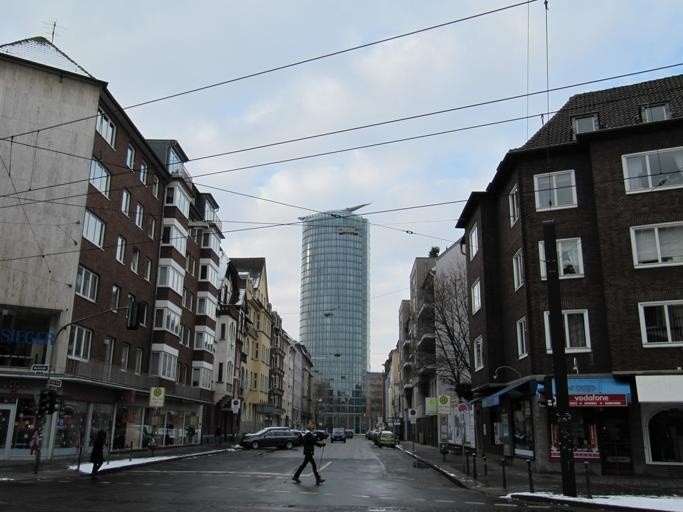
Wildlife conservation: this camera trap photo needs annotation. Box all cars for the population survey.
[365,426,400,447]
[330,427,353,442]
[232,423,329,449]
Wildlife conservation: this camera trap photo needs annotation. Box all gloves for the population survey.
[321,443,325,446]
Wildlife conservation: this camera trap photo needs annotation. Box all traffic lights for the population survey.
[538,376,553,410]
[37,388,62,416]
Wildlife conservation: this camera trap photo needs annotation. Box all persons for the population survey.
[292,425,326,484]
[29,430,41,455]
[89,430,108,481]
[215,424,223,446]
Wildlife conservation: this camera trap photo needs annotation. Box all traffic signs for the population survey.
[48,378,61,387]
[30,363,49,372]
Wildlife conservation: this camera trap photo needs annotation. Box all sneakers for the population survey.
[316,479,324,484]
[292,475,300,482]
